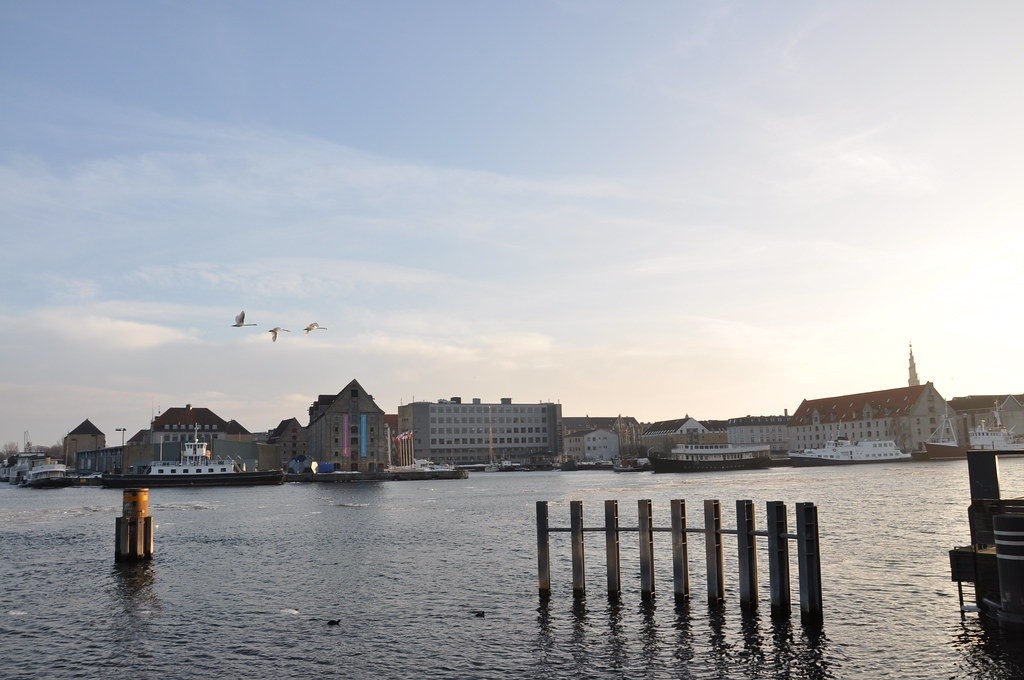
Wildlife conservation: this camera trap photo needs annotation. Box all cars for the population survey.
[91,473,103,478]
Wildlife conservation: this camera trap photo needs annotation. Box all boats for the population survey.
[968,398,1024,454]
[0,452,74,489]
[577,459,614,469]
[513,466,530,471]
[102,437,284,488]
[650,442,774,472]
[785,434,913,465]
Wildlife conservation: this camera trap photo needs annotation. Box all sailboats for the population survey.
[610,414,649,472]
[922,397,965,460]
[482,405,501,472]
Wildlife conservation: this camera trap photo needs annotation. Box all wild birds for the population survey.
[303,322,329,336]
[268,326,292,343]
[330,618,342,626]
[475,610,485,618]
[231,310,258,328]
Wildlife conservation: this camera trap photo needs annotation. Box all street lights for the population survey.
[92,435,98,453]
[72,438,78,451]
[116,428,126,446]
